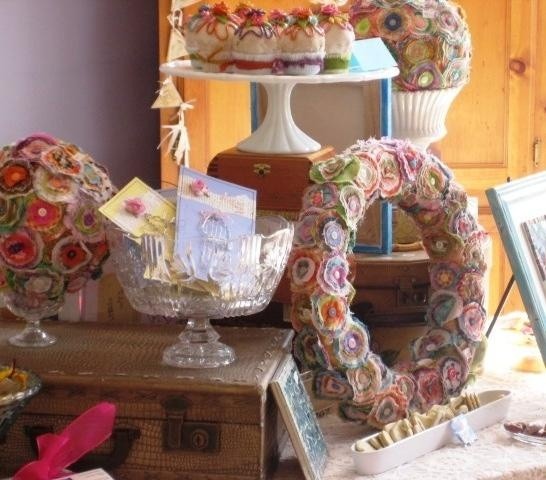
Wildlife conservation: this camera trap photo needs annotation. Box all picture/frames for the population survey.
[486,169,546,370]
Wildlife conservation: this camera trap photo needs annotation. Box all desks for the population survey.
[278,310,546,479]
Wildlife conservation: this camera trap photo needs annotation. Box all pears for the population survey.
[0,358,20,396]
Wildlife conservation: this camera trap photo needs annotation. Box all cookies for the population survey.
[355,391,481,451]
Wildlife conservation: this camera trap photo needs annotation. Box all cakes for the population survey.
[185,1,355,76]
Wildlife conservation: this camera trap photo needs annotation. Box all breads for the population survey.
[503,418,546,439]
